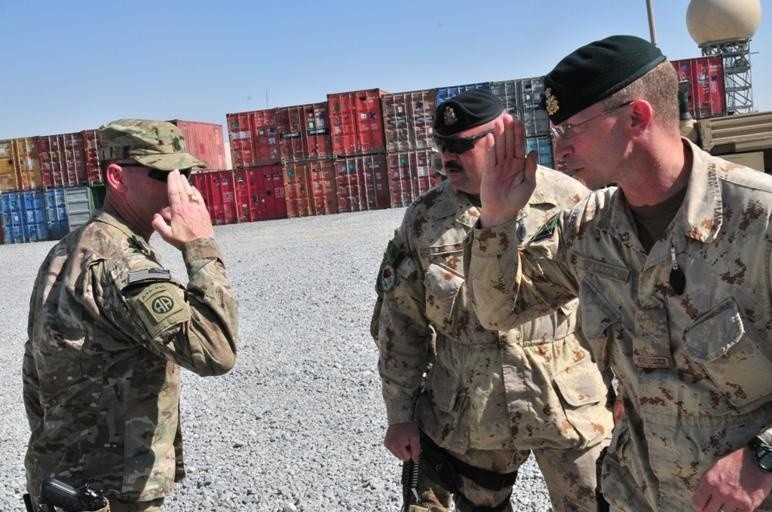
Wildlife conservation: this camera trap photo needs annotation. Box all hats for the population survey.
[97,117,207,172]
[538,35,667,127]
[434,87,503,136]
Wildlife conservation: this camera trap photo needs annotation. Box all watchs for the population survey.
[745,438,770,473]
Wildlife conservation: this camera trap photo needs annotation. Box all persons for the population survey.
[371,87,625,512]
[22,119,238,511]
[467,35,771,512]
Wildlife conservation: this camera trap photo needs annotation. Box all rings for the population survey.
[189,194,200,204]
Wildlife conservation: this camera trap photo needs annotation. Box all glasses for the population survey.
[111,163,192,182]
[546,99,634,142]
[431,128,496,156]
